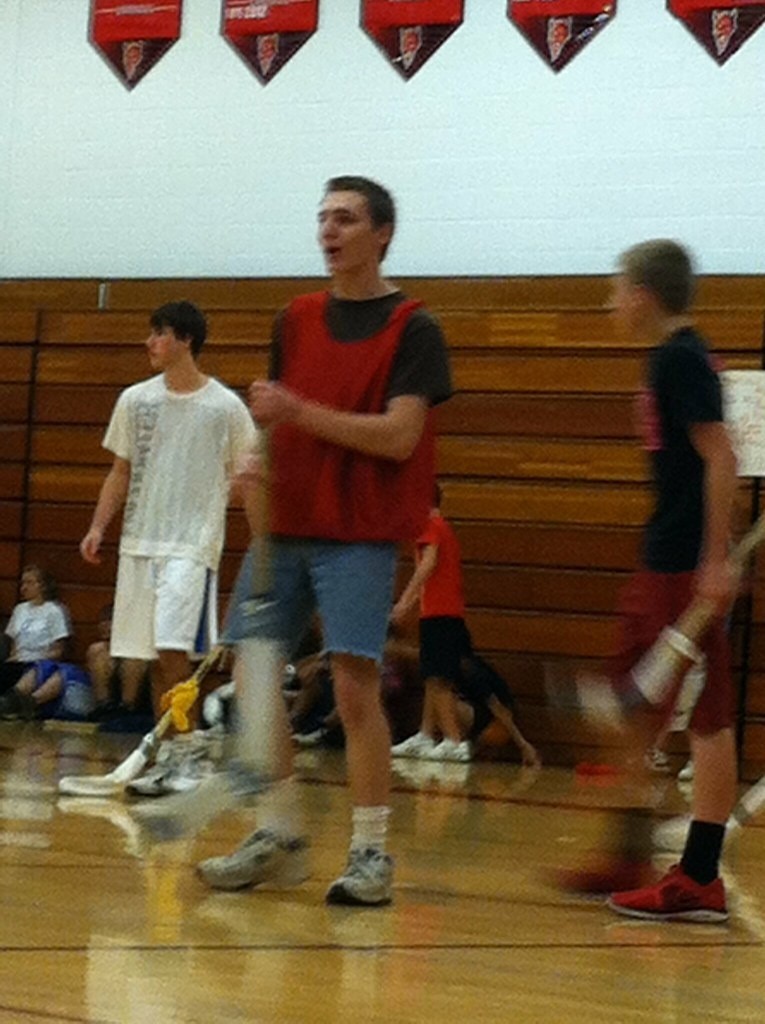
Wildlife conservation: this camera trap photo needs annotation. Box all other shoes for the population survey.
[12,685,35,720]
[0,698,17,719]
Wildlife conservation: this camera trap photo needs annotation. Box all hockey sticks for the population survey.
[60,646,229,797]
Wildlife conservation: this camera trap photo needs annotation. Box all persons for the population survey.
[193,174,457,908]
[538,240,743,923]
[0,479,541,768]
[81,298,263,799]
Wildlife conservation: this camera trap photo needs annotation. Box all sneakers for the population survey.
[428,741,470,761]
[198,828,312,890]
[389,737,434,757]
[326,848,393,905]
[608,864,730,921]
[163,730,228,790]
[124,739,175,796]
[556,859,651,900]
